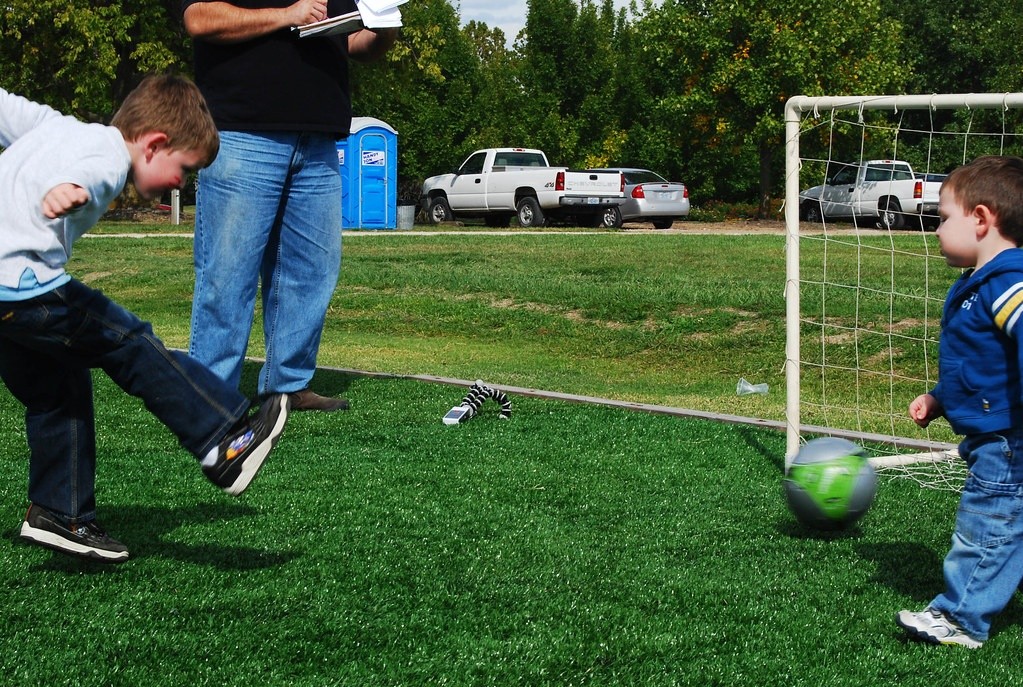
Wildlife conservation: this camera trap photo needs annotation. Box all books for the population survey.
[296,10,363,39]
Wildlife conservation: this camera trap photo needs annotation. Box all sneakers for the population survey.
[202,392,292,497]
[19,503,130,562]
[895,607,983,651]
[290,388,348,410]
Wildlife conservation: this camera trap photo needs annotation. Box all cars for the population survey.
[547,168,690,229]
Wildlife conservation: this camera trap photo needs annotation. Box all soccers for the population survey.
[786,436,876,529]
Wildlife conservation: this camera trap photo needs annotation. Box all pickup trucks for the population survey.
[419,148,628,228]
[799,160,950,230]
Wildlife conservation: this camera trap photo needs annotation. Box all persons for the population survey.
[894,154,1022,649]
[0,73,287,563]
[178,0,401,413]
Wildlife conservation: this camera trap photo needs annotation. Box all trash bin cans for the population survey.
[335,117,399,229]
